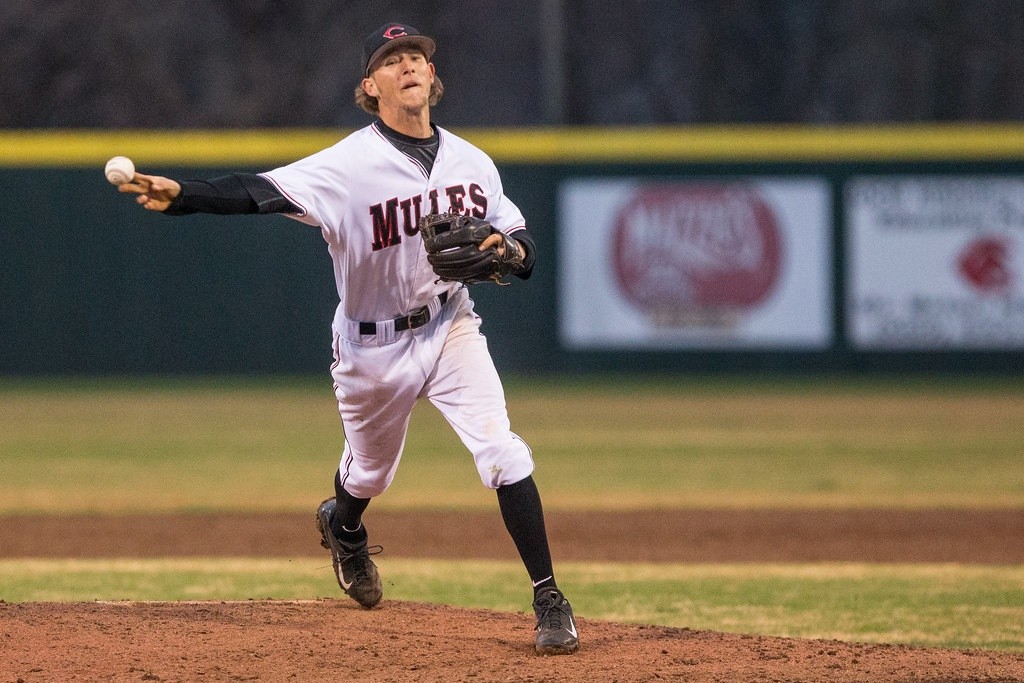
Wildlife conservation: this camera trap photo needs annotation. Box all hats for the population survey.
[361,22,435,78]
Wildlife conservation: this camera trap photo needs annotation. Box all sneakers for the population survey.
[318,497,381,609]
[533,587,579,655]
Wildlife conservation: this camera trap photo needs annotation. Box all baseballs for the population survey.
[105,155,136,186]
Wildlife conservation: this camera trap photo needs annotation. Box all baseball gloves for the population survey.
[418,212,521,286]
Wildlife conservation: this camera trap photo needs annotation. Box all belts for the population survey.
[359,291,447,334]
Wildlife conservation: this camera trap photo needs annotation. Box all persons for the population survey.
[120,23,580,655]
